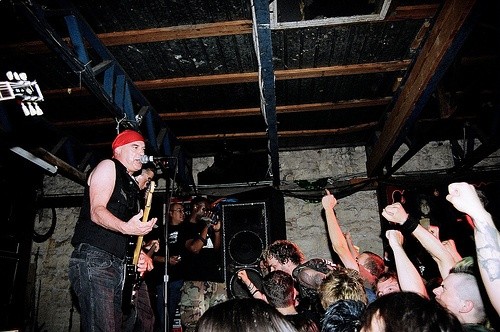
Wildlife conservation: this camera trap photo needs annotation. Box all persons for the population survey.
[196,182,500,332]
[151,197,226,332]
[68,130,159,332]
[177,197,228,332]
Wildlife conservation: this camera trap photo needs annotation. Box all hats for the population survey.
[112,129,144,149]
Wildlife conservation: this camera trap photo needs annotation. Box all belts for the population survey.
[76,237,124,260]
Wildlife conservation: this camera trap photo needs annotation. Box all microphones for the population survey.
[140,155,177,164]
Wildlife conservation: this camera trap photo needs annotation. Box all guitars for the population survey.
[137,237,160,290]
[121,180,156,316]
[0,70,46,117]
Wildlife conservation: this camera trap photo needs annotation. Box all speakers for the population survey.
[222,201,287,300]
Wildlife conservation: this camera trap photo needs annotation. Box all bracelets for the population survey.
[403,214,418,233]
[249,285,255,291]
[251,290,259,295]
[215,229,220,232]
[196,234,208,246]
[247,282,251,287]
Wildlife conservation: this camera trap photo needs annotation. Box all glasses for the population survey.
[170,208,185,212]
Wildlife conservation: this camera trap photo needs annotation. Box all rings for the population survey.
[444,243,446,245]
[176,260,178,263]
